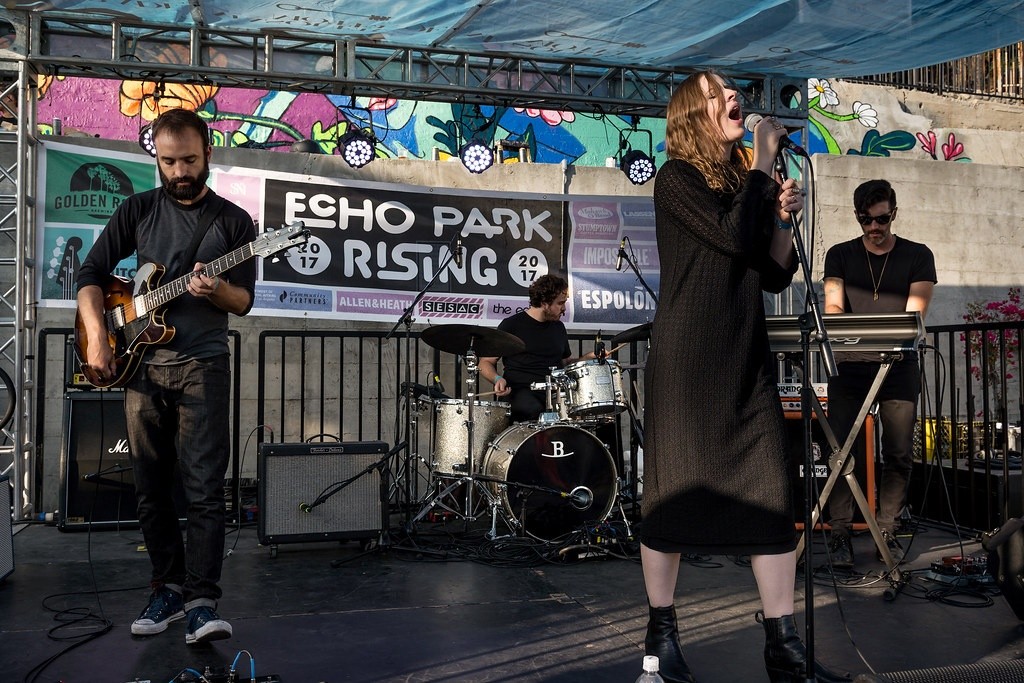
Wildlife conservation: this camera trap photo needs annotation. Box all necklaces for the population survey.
[866,239,892,302]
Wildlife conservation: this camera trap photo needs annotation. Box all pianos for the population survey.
[763,310,928,586]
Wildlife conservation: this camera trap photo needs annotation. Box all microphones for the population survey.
[572,490,591,507]
[456,232,462,269]
[616,238,626,271]
[593,331,601,355]
[80,473,96,481]
[298,503,311,513]
[433,371,446,393]
[743,113,810,158]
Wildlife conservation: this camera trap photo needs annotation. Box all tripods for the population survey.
[328,250,516,569]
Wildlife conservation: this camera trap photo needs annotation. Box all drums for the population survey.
[431,398,514,480]
[478,420,620,545]
[555,378,615,427]
[557,357,628,420]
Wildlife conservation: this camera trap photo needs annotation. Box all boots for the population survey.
[645,605,697,683]
[753,608,854,683]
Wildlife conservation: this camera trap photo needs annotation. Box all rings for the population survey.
[791,195,799,202]
[792,178,797,182]
[791,187,800,195]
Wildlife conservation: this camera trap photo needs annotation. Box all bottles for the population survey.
[635,656,665,683]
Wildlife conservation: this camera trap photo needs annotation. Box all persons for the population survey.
[637,69,854,683]
[477,274,609,427]
[823,179,938,567]
[75,108,256,645]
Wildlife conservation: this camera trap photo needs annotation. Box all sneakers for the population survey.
[186,606,233,645]
[876,531,907,562]
[130,587,187,634]
[827,533,855,565]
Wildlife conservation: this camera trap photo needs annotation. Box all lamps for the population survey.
[458,105,496,175]
[336,93,377,169]
[138,82,183,158]
[619,115,656,186]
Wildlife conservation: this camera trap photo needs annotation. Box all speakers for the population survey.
[0,474,15,581]
[258,441,389,546]
[57,394,189,533]
[853,657,1024,683]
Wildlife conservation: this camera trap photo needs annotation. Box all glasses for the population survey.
[856,209,894,225]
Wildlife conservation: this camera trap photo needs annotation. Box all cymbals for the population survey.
[610,321,653,343]
[420,323,528,359]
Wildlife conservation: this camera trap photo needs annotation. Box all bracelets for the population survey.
[775,215,792,230]
[493,375,502,385]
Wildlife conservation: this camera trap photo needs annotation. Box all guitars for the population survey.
[74,220,311,390]
